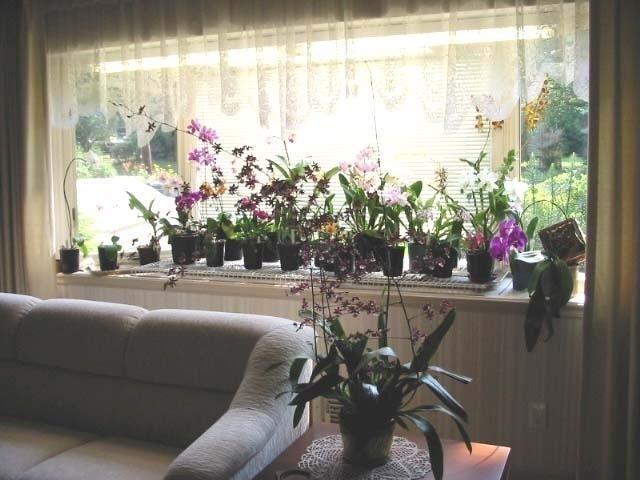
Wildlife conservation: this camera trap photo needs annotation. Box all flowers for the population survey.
[203,228,223,242]
[172,191,209,235]
[406,190,438,246]
[490,219,527,264]
[307,168,344,243]
[377,178,413,244]
[200,179,242,240]
[102,98,472,480]
[337,145,411,237]
[430,166,471,248]
[426,72,551,253]
[266,129,301,246]
[212,143,273,241]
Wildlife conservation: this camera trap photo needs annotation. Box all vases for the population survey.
[509,255,527,290]
[333,408,396,468]
[314,251,335,273]
[276,241,300,271]
[205,242,225,268]
[380,243,405,277]
[241,240,265,270]
[408,242,432,275]
[354,229,380,270]
[223,239,243,261]
[431,245,456,278]
[466,250,493,284]
[171,234,202,264]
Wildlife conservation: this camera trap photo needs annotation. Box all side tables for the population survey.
[254,421,513,480]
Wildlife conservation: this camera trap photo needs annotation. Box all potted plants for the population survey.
[126,187,166,265]
[519,164,586,264]
[58,235,89,274]
[97,234,121,272]
[524,236,579,354]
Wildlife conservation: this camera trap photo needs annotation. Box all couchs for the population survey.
[2,290,316,480]
